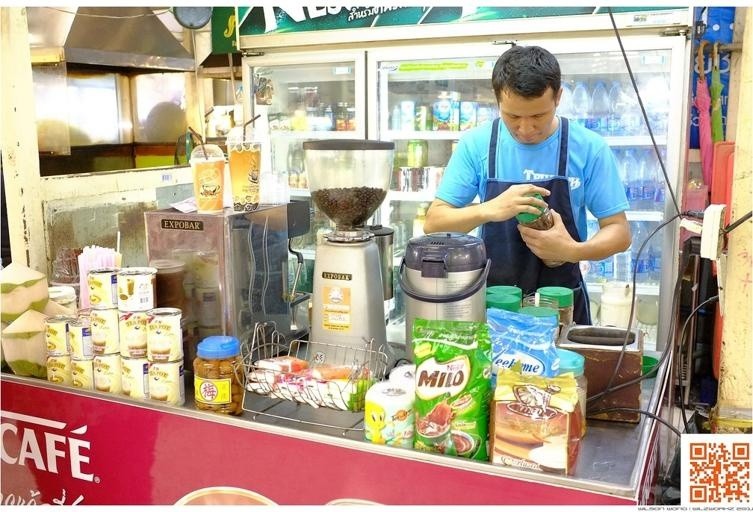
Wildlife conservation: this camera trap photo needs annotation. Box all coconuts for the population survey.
[1,262,75,378]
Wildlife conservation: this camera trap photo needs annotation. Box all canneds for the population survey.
[516,193,566,268]
[397,139,437,192]
[44,267,246,416]
[486,285,575,317]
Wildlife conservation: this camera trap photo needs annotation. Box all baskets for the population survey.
[230,320,387,437]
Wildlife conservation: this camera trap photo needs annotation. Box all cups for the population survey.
[190,129,264,214]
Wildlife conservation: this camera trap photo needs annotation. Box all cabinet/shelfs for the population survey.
[368,32,692,349]
[240,50,367,262]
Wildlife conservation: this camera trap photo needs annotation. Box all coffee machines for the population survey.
[303,138,392,376]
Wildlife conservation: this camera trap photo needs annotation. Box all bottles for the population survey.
[486,79,667,343]
[391,104,401,129]
[412,208,428,237]
[287,142,304,185]
[288,87,355,131]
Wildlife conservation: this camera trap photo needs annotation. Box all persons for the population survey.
[423,46,630,325]
